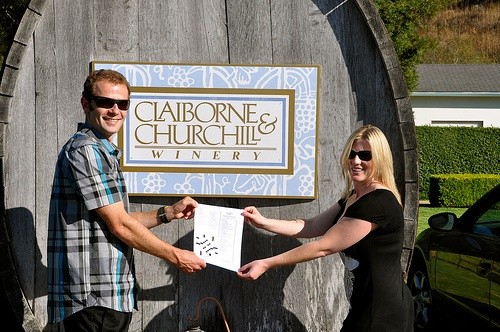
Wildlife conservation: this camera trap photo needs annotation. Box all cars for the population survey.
[406,182,500,332]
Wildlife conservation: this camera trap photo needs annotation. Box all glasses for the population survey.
[89,95,131,110]
[348,150,372,161]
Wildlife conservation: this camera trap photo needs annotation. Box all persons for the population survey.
[48,69,206,331]
[237,125,415,332]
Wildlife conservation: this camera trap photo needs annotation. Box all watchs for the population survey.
[158,206,171,224]
